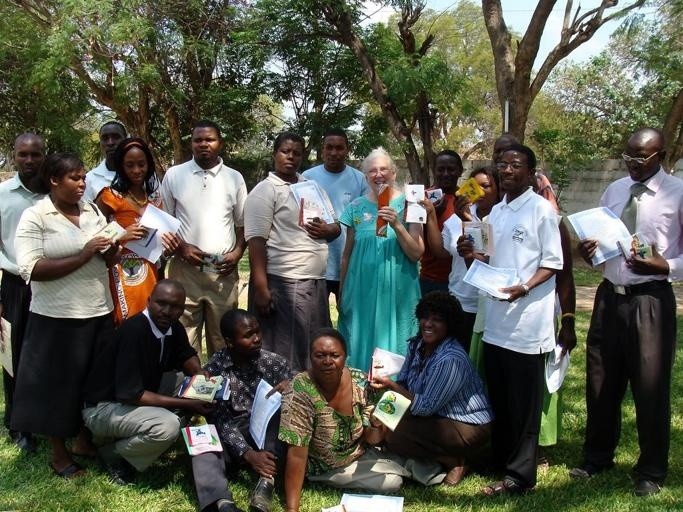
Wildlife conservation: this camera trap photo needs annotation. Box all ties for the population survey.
[620,183,647,233]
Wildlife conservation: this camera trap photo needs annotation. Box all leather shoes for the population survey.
[537,452,549,472]
[249,478,276,512]
[216,500,238,511]
[567,462,609,480]
[633,478,663,497]
[108,462,138,485]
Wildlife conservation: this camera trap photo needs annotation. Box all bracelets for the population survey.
[561,313,576,319]
[522,284,529,295]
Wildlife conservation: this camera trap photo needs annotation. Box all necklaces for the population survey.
[122,182,149,207]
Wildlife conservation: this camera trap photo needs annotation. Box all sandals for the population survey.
[475,479,531,498]
[443,463,464,485]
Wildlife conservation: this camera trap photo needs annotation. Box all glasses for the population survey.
[620,147,659,167]
[494,160,532,171]
[363,165,394,176]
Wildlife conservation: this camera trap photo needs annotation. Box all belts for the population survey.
[602,278,658,297]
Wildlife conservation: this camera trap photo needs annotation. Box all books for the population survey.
[181,424,224,456]
[90,221,127,254]
[617,231,654,263]
[462,221,494,258]
[178,375,231,403]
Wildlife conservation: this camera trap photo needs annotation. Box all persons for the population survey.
[338,147,426,454]
[367,291,495,487]
[454,133,576,469]
[83,120,128,202]
[83,279,232,487]
[417,166,502,353]
[10,154,121,476]
[302,127,373,293]
[182,309,294,512]
[277,326,448,512]
[161,121,248,367]
[244,132,342,372]
[0,134,45,452]
[419,150,462,297]
[456,144,564,497]
[569,128,683,496]
[93,138,183,334]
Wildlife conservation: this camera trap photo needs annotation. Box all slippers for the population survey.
[71,442,98,458]
[48,458,87,480]
